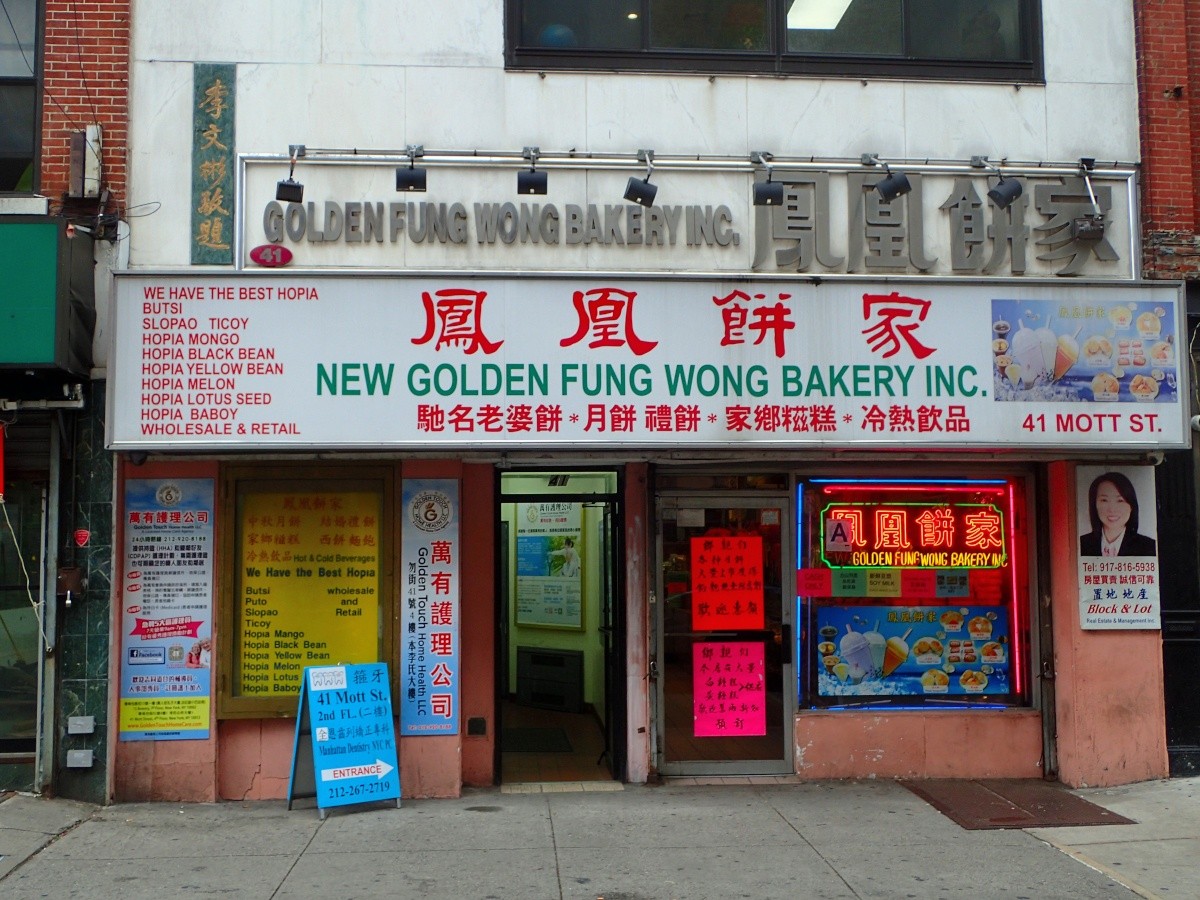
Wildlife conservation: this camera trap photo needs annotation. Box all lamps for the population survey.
[396,145,427,191]
[518,147,548,194]
[860,154,912,204]
[749,152,783,205]
[1071,163,1104,239]
[624,150,658,206]
[276,145,307,203]
[971,156,1022,208]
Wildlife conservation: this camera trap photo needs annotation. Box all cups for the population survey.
[817,626,907,687]
[992,321,1075,392]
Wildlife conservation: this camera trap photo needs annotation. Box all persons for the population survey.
[1080,472,1156,557]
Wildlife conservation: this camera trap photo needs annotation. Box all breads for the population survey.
[969,616,990,633]
[1083,340,1113,357]
[1130,375,1157,395]
[1091,373,1119,395]
[960,670,985,685]
[921,669,948,686]
[914,640,941,654]
